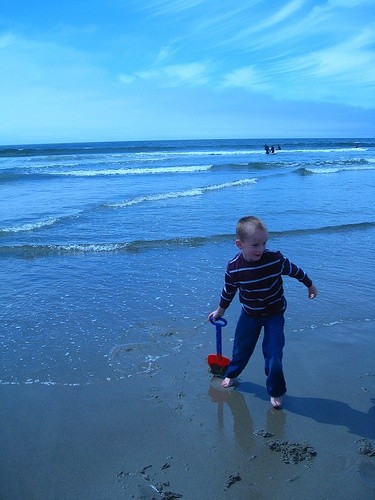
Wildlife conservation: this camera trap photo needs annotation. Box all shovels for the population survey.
[207,317,232,375]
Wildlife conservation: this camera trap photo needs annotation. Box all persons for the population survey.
[264,144,269,154]
[276,144,281,150]
[208,216,317,406]
[271,144,274,153]
